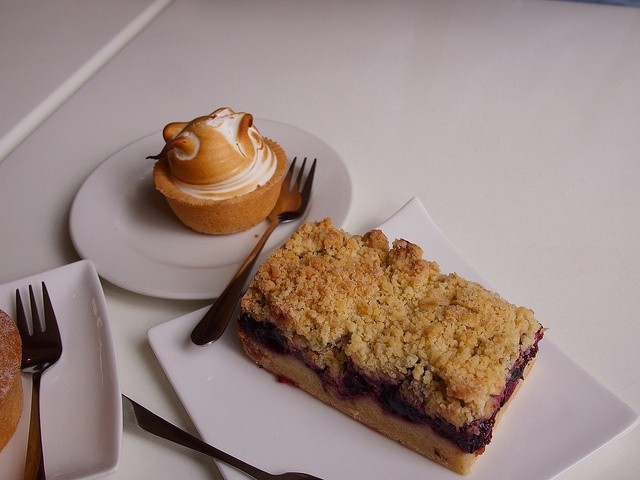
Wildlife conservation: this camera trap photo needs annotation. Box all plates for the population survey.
[0,259,123,480]
[68,116,352,301]
[145,196,640,480]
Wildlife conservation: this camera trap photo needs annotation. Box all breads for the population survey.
[235,217,549,477]
[0,307,24,454]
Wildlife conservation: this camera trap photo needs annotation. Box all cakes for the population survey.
[144,105,288,235]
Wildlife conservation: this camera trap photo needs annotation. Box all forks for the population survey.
[190,156,317,348]
[16,281,62,480]
